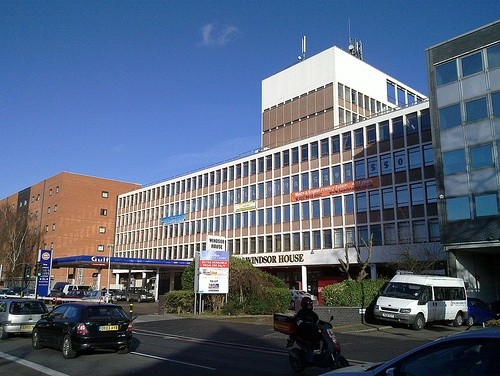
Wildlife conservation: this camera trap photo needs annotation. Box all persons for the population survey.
[294,297,323,362]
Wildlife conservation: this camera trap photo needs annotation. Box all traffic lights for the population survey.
[34,263,43,277]
[24,263,32,279]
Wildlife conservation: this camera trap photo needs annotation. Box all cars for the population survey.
[288,289,318,310]
[0,286,27,306]
[318,326,500,376]
[125,287,155,303]
[21,289,36,297]
[52,282,95,299]
[466,297,498,327]
[32,302,133,360]
[101,288,127,301]
[0,298,49,340]
[44,290,66,304]
[82,290,117,304]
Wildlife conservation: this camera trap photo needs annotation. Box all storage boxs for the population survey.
[274,313,297,333]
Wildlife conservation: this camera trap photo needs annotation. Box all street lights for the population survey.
[107,244,114,293]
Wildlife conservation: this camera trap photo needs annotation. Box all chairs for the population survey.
[92,309,102,315]
[24,303,31,309]
[13,304,21,310]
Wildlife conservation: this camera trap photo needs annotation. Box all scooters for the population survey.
[285,315,350,374]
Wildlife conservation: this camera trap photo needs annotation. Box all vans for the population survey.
[373,274,469,331]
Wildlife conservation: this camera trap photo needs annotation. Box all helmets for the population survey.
[300,297,313,311]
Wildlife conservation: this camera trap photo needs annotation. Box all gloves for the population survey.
[305,322,314,328]
[324,322,332,329]
